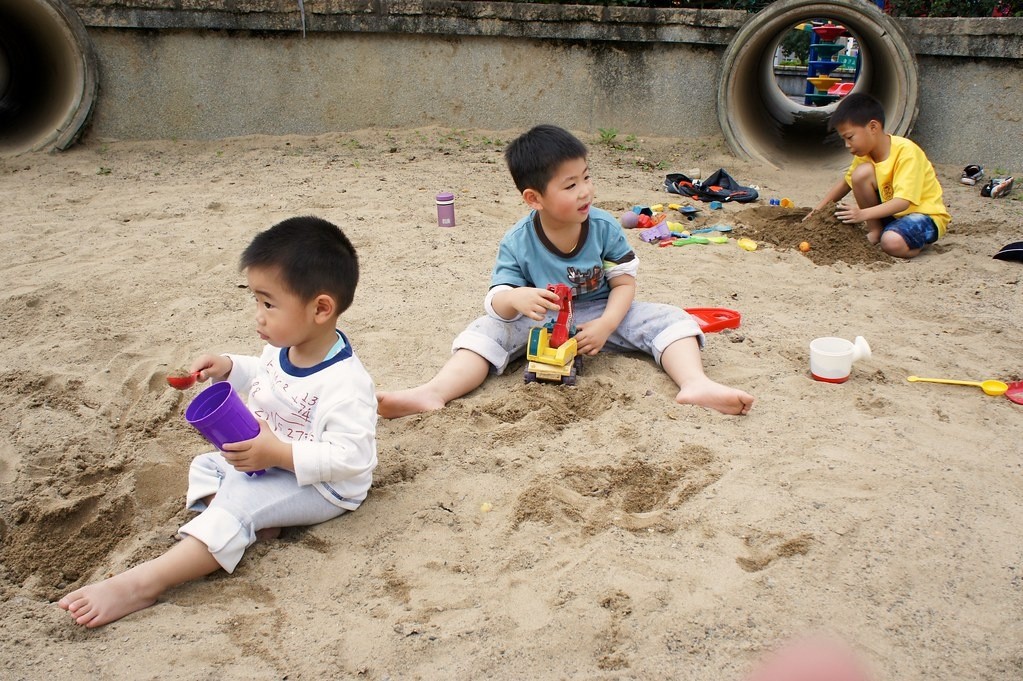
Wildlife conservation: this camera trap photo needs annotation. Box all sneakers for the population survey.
[959,163,983,185]
[982,177,1014,198]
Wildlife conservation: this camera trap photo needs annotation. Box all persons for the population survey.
[802,93,953,258]
[58,216,378,630]
[376,124,755,418]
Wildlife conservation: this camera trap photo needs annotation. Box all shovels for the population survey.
[671,238,709,247]
[692,224,732,234]
[668,202,703,215]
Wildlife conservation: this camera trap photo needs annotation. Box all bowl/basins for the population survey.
[810,337,853,383]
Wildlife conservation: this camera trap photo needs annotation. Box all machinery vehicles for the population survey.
[522,281,583,385]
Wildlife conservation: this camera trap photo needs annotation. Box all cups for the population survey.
[186,381,276,477]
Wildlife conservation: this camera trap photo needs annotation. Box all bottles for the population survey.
[436,193,456,227]
